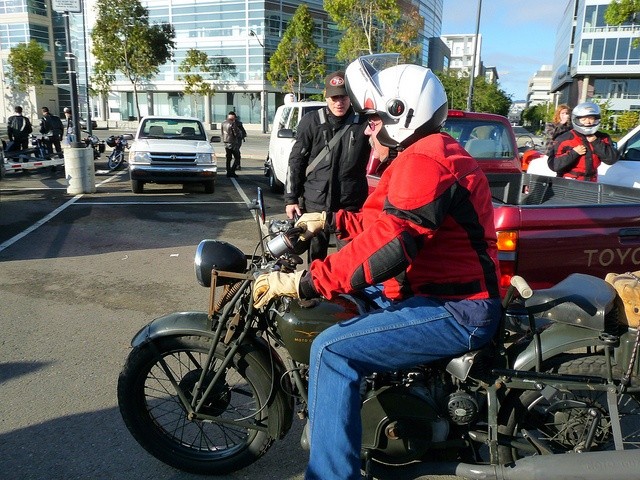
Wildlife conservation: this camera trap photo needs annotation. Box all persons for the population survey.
[37,107,63,159]
[229,111,247,169]
[547,101,616,181]
[222,114,245,176]
[64,106,76,132]
[547,104,569,155]
[7,105,31,163]
[286,72,370,266]
[254,51,504,480]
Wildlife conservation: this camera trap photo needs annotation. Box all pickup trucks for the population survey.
[78,117,97,128]
[363,109,640,299]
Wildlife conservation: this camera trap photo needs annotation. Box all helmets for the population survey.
[345,53,448,150]
[106,137,115,147]
[571,103,601,135]
[267,218,310,256]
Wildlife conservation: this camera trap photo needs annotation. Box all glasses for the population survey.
[366,116,382,129]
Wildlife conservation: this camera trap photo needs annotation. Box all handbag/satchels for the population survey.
[606,269,640,329]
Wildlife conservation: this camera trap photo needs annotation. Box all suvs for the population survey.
[265,100,329,193]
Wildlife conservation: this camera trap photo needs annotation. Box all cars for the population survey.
[122,116,222,193]
[527,124,640,188]
[510,126,546,148]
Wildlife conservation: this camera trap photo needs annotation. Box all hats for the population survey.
[325,71,347,97]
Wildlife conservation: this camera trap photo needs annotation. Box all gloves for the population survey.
[294,211,326,241]
[251,269,307,309]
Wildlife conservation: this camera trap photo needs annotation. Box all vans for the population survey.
[60,119,72,128]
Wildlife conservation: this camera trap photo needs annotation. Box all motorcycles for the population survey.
[106,134,131,171]
[29,129,53,160]
[116,186,640,479]
[82,129,106,160]
